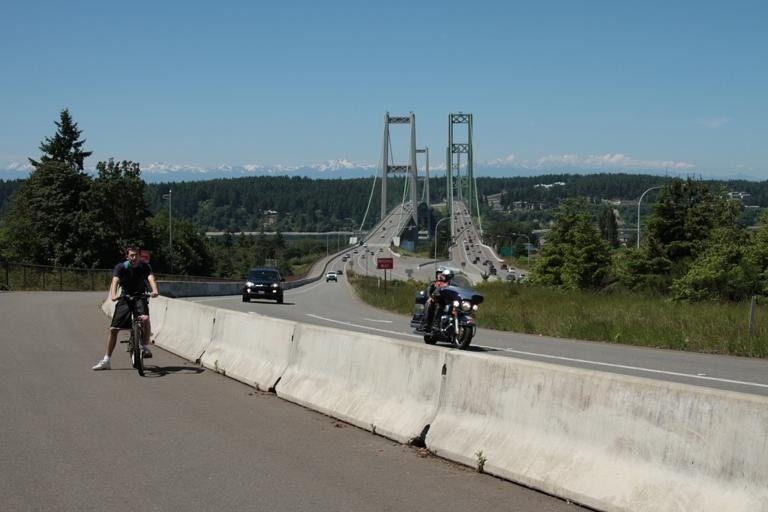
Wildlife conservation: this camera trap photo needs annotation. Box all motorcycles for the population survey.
[410,284,484,350]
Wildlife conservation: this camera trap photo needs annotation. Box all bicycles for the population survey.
[117,291,152,377]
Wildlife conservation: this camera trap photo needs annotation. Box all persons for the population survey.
[415,267,446,334]
[91,244,160,370]
[431,269,456,328]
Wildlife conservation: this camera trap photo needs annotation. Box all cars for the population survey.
[242,267,285,303]
[489,264,525,280]
[326,270,337,282]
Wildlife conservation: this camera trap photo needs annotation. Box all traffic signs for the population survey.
[376,258,392,269]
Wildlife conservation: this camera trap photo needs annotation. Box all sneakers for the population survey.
[92,360,111,370]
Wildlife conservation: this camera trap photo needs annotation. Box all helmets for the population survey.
[436,267,454,275]
